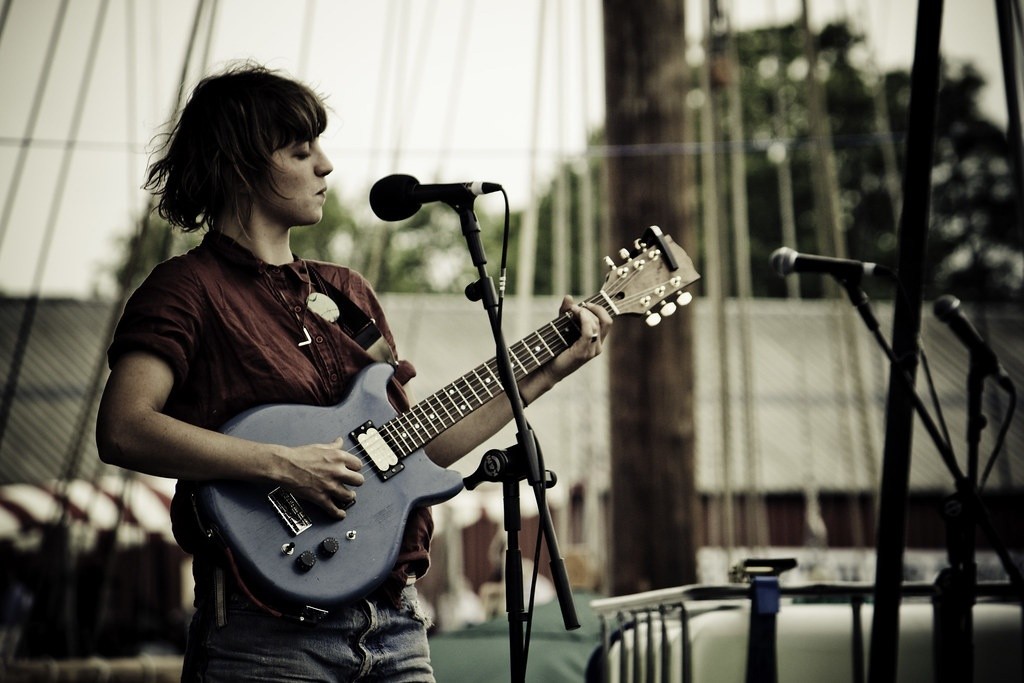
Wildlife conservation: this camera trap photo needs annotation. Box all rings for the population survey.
[591,334,597,343]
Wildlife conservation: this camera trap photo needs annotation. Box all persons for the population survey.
[96,73,614,681]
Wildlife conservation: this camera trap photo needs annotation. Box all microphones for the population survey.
[370,174,503,222]
[933,295,1016,395]
[770,247,879,276]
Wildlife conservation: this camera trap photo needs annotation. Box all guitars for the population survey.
[186,223,706,628]
